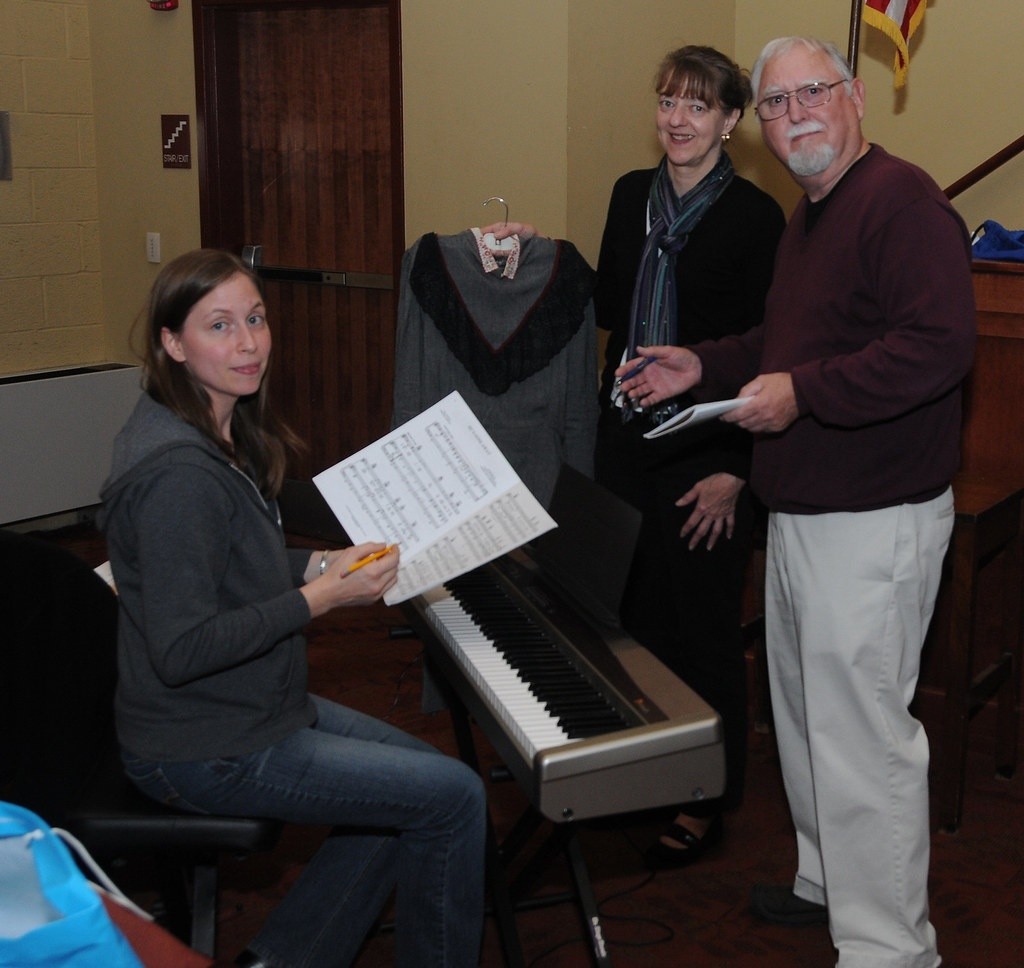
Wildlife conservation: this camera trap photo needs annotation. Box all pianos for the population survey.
[402,543,728,825]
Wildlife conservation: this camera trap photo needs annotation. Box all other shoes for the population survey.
[645,814,724,865]
[752,886,827,926]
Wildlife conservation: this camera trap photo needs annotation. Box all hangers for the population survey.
[482,196,517,257]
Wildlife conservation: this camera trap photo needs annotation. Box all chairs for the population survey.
[0,503,280,962]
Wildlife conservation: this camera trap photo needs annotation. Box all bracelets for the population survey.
[320,547,331,574]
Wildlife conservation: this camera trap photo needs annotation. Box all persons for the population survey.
[99,246,494,968]
[613,29,981,967]
[478,45,791,869]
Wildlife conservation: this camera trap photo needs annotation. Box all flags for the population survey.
[860,0,928,91]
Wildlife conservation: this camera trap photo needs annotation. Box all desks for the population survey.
[745,260,1024,845]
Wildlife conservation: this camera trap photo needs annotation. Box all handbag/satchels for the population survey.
[969,220,1024,261]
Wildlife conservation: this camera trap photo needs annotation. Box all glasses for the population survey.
[754,78,850,120]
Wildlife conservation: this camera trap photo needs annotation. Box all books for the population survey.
[643,395,756,441]
